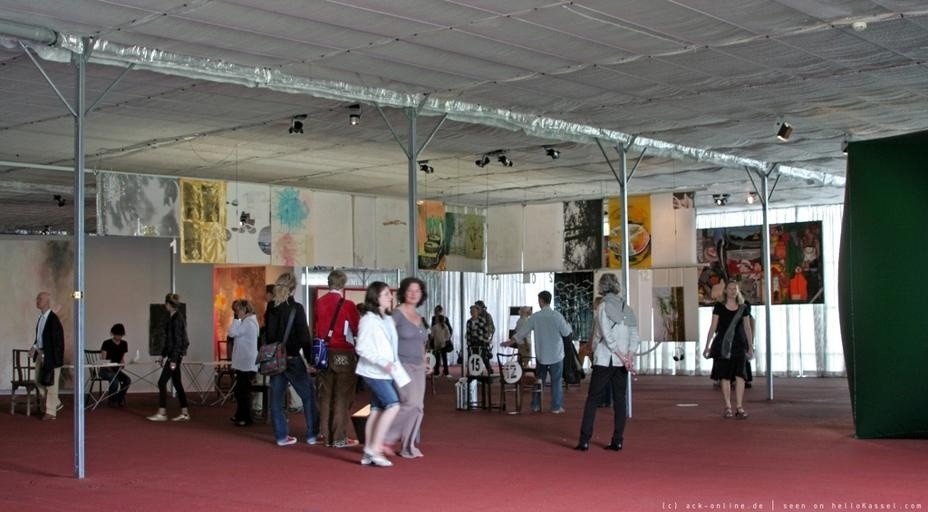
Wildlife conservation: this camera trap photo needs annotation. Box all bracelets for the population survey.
[623,357,626,363]
[748,344,754,349]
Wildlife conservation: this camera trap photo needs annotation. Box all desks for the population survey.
[60,361,127,411]
[127,356,240,410]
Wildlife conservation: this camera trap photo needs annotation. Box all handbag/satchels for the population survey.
[707,326,735,360]
[446,340,454,352]
[255,341,288,375]
[37,365,55,385]
[310,338,329,370]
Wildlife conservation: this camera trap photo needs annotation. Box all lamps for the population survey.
[415,136,565,174]
[284,113,309,137]
[347,110,363,128]
[707,120,850,209]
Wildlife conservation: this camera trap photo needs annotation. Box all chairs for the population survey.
[83,349,127,408]
[461,338,546,414]
[216,340,240,401]
[10,349,45,420]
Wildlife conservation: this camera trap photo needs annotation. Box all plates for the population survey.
[608,222,649,256]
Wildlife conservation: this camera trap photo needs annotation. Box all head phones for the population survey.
[673,346,685,362]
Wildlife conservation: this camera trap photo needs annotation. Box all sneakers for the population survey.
[170,413,191,421]
[146,414,168,421]
[40,413,56,422]
[277,436,297,446]
[307,434,324,444]
[362,447,393,467]
[360,455,372,464]
[324,440,333,447]
[332,438,359,448]
[56,403,64,411]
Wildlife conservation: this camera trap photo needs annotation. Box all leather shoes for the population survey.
[574,443,589,451]
[604,442,622,451]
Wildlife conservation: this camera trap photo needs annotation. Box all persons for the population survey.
[146,293,192,423]
[729,300,755,389]
[99,323,130,408]
[28,292,64,422]
[227,268,573,469]
[702,280,756,420]
[572,270,639,452]
[585,297,612,407]
